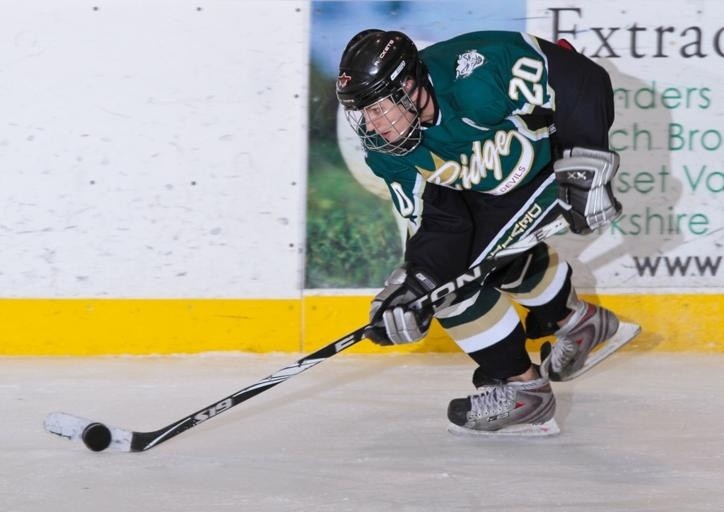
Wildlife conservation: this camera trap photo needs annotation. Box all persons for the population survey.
[335,28,623,433]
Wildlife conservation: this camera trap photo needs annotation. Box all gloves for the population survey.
[365,262,447,346]
[553,147,623,235]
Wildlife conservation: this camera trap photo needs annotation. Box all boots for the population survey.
[447,286,620,432]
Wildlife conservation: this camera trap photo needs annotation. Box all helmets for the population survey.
[336,29,428,110]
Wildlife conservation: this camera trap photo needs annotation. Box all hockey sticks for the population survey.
[48,216,570,452]
